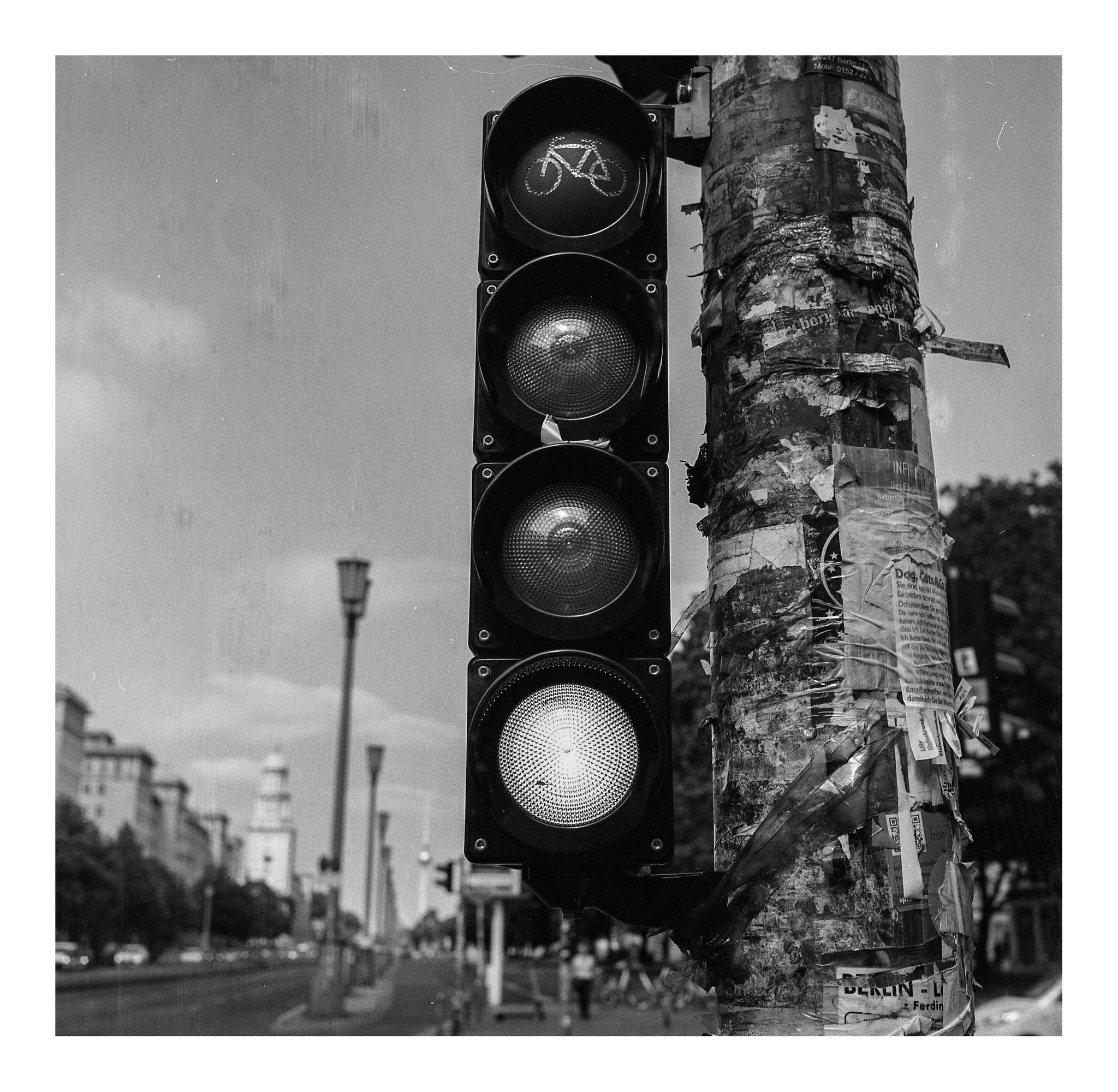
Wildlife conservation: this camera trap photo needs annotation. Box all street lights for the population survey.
[315,556,376,1018]
[360,742,397,981]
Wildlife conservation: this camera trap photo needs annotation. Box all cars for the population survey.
[113,944,149,966]
[178,946,207,965]
[55,941,87,972]
[973,971,1063,1036]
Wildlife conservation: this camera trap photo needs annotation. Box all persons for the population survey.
[571,941,596,1021]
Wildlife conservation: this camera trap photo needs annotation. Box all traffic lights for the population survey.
[435,861,454,891]
[461,73,675,870]
